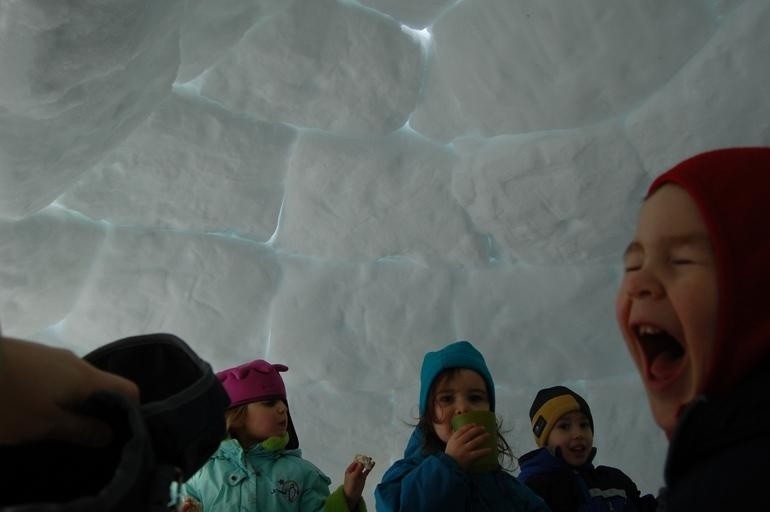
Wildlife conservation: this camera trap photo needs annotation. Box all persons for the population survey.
[514,385,657,512]
[614,146,769,512]
[0,335,141,447]
[373,340,552,512]
[170,359,376,512]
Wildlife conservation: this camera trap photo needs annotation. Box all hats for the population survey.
[416,338,497,418]
[527,385,592,452]
[213,360,300,452]
[639,144,770,433]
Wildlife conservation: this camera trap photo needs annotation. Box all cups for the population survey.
[452,412,499,465]
